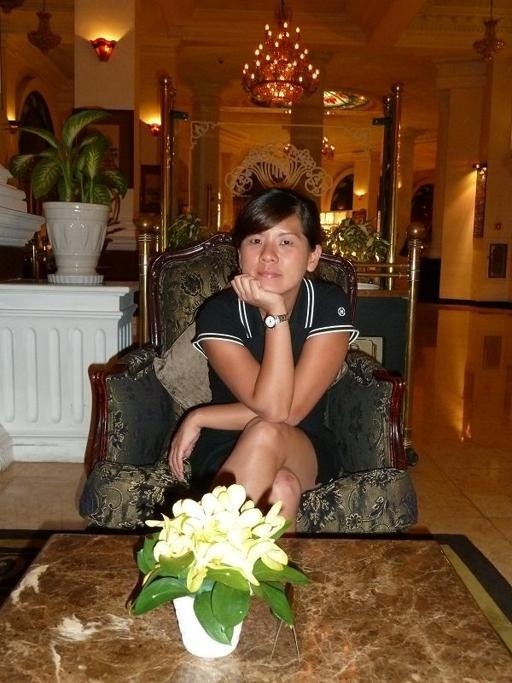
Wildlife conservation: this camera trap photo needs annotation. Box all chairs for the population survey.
[79,230,418,535]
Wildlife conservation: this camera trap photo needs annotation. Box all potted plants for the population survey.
[6,107,127,285]
[323,218,392,290]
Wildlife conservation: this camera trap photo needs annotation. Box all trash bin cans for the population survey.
[419,257,441,304]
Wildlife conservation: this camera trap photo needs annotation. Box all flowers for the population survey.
[128,484,310,645]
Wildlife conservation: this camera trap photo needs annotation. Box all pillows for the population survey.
[152,321,211,409]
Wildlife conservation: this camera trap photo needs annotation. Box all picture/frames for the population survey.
[139,164,162,214]
[488,242,508,278]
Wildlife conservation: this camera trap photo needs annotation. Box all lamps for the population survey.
[90,37,118,63]
[239,0,320,109]
[26,0,62,57]
[472,0,505,64]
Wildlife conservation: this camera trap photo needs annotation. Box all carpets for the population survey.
[0,520,512,683]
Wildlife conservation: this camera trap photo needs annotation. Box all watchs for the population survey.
[260,313,288,329]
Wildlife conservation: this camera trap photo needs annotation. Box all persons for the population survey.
[168,186,359,536]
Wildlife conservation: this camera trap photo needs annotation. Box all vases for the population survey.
[171,596,244,657]
